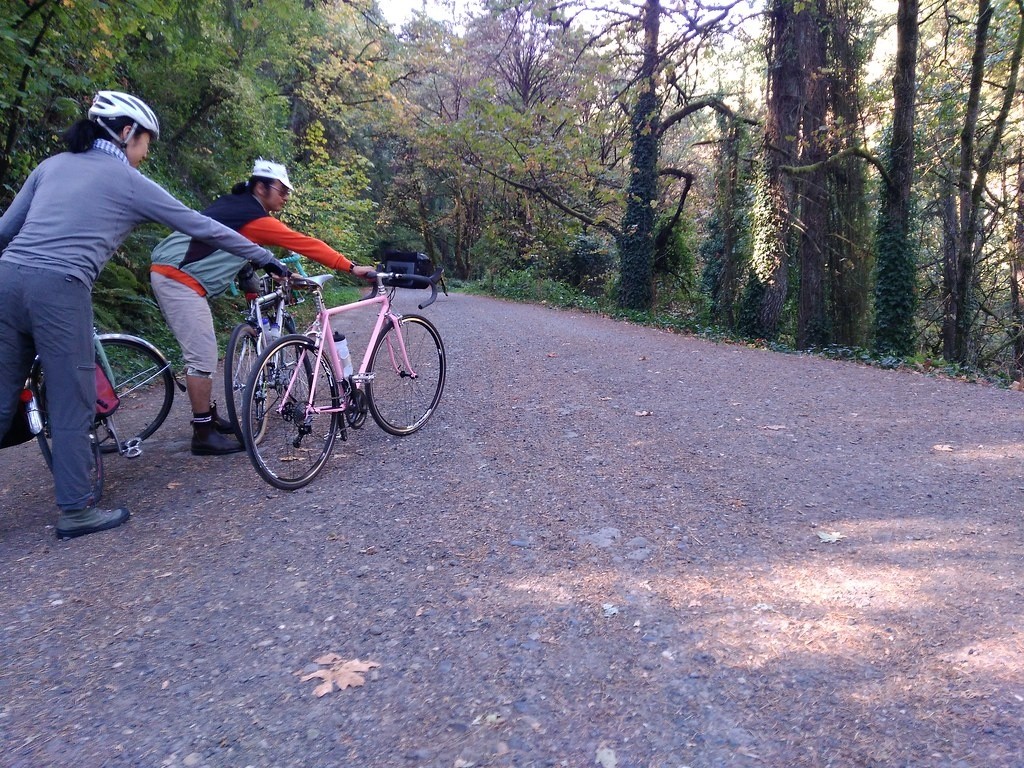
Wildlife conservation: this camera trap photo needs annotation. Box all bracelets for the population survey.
[349,264,354,273]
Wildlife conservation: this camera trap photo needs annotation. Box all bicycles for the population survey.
[222,257,320,448]
[239,266,449,490]
[22,321,188,510]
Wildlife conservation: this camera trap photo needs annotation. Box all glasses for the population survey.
[269,184,290,197]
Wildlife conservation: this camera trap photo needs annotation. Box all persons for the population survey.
[0,89,305,540]
[150,157,376,456]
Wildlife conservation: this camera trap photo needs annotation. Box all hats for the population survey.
[251,159,295,192]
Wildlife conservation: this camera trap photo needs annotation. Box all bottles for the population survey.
[261,316,273,345]
[271,323,280,355]
[333,331,353,378]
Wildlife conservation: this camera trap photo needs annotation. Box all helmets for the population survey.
[87,90,159,141]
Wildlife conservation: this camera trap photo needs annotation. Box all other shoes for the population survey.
[189,420,243,455]
[210,399,234,435]
[53,503,130,540]
